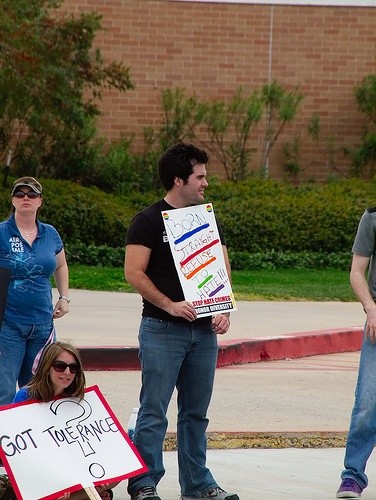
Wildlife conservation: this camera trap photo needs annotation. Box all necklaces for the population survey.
[21,230,36,236]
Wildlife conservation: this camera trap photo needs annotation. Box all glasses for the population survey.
[50,361,79,372]
[12,190,40,198]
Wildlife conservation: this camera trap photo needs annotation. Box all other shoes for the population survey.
[100,489,113,500]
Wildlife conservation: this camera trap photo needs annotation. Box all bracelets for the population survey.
[59,296,70,303]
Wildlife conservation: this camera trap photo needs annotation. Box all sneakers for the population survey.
[336,478,362,498]
[181,486,239,500]
[131,486,161,500]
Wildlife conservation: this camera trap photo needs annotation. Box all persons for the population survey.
[335,206,376,499]
[0,177,70,405]
[124,144,239,500]
[12,342,113,500]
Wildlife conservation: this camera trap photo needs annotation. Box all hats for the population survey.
[11,177,42,196]
